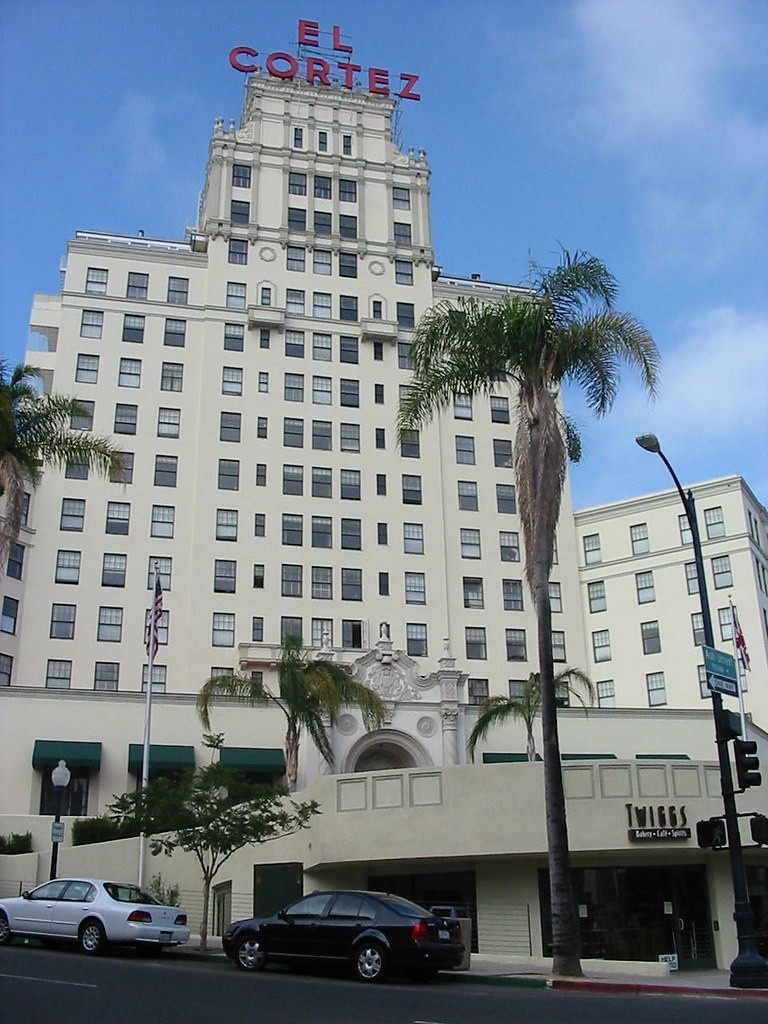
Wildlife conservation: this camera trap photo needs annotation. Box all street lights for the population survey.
[634,428,768,991]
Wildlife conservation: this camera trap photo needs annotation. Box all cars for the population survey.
[0,877,192,960]
[221,888,466,984]
